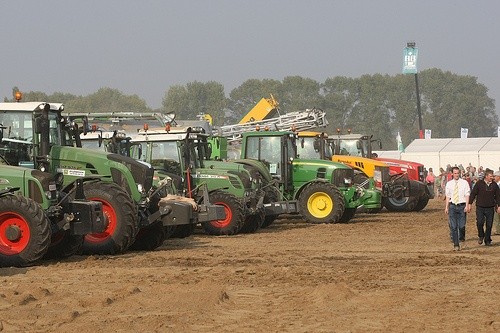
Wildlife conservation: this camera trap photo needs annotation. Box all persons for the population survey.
[428,163,500,235]
[467,168,500,245]
[444,167,471,251]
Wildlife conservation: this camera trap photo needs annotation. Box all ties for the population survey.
[453,182,460,205]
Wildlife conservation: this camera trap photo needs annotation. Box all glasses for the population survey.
[486,189,492,192]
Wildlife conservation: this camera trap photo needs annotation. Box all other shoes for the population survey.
[461,241,466,249]
[478,238,492,246]
[452,246,460,251]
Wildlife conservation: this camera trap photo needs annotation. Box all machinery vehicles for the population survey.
[0,92,435,268]
